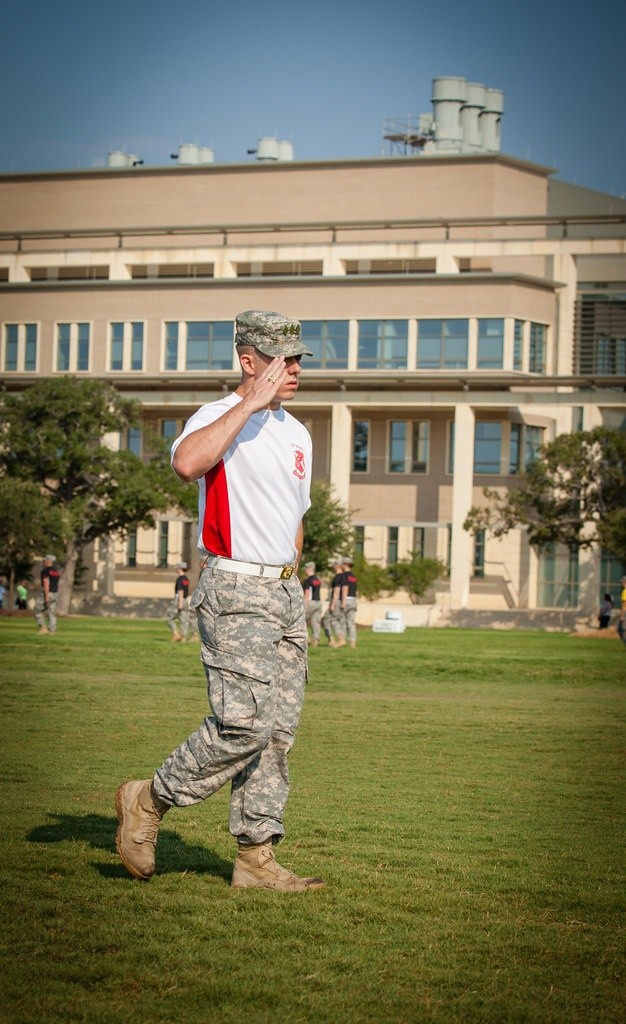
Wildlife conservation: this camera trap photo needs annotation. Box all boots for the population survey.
[115,779,170,880]
[231,835,325,892]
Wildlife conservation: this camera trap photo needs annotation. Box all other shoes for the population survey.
[37,625,48,636]
[306,635,355,649]
[168,631,200,644]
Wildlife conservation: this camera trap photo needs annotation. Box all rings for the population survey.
[269,376,273,382]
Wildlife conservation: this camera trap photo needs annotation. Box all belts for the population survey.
[207,557,296,580]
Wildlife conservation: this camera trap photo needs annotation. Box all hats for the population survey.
[43,555,56,562]
[342,558,352,564]
[235,310,314,358]
[334,560,342,565]
[302,562,315,569]
[201,556,209,562]
[175,562,187,569]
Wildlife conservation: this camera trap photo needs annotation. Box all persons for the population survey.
[166,563,190,643]
[188,556,209,642]
[301,561,323,647]
[323,557,358,648]
[16,581,28,611]
[598,594,613,628]
[0,576,8,609]
[115,309,326,894]
[33,555,60,634]
[616,576,626,642]
